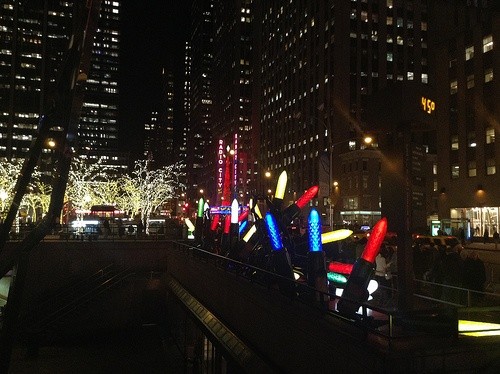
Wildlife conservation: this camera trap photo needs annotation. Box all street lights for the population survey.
[324,136,377,231]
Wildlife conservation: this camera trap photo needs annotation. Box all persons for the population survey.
[326,223,500,305]
[7,216,164,240]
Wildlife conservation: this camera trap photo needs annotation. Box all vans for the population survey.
[417,234,464,257]
